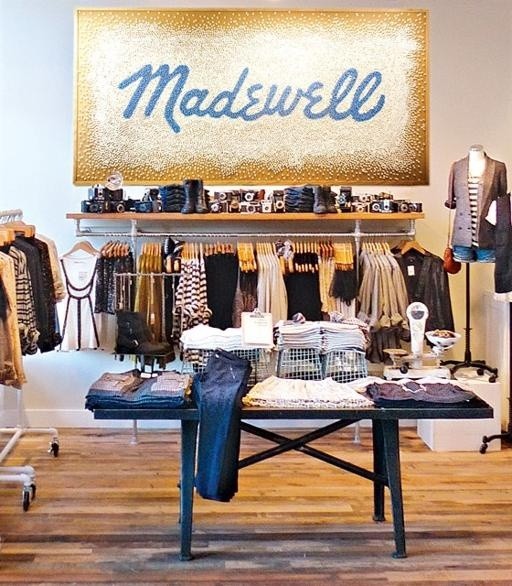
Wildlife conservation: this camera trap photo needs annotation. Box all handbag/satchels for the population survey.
[443,247,461,274]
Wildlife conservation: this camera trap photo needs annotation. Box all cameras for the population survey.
[81,185,158,212]
[337,187,422,213]
[211,190,285,213]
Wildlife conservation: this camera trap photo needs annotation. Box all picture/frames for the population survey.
[71,6,429,186]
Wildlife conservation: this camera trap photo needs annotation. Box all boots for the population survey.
[313,185,327,214]
[194,179,209,213]
[324,186,337,213]
[181,180,195,214]
[116,310,171,354]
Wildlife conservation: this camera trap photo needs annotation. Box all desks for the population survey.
[94,367,495,563]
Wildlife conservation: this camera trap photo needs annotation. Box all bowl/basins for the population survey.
[425,330,461,347]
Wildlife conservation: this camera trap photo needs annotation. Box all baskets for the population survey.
[323,351,367,383]
[229,349,258,386]
[279,348,323,381]
[182,348,215,378]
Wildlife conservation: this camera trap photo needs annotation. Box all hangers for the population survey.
[1,208,35,248]
